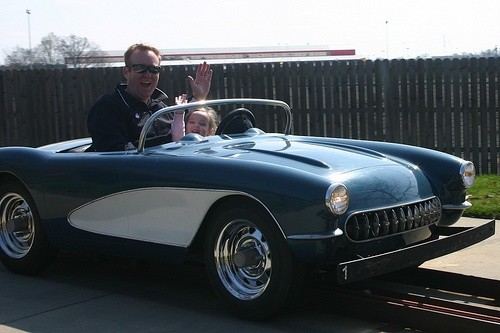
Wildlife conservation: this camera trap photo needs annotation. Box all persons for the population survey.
[85,42,213,155]
[170,94,216,147]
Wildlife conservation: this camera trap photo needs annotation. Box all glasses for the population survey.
[126,63,161,74]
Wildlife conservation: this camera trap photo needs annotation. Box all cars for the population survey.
[0,98,496,320]
